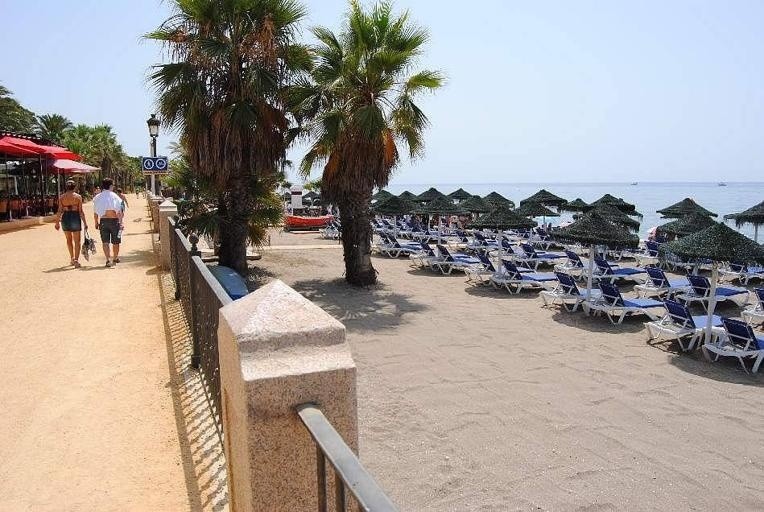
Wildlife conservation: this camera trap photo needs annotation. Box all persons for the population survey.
[115,188,128,225]
[54,181,88,270]
[91,178,123,267]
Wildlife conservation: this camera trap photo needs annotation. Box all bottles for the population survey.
[116,226,122,239]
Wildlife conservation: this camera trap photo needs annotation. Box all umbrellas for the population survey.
[0,136,104,175]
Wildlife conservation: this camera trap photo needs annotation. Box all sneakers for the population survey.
[69,256,121,268]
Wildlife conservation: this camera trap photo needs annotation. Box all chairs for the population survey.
[370,215,764,377]
[319,213,342,240]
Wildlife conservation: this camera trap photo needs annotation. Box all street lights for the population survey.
[146,114,161,195]
